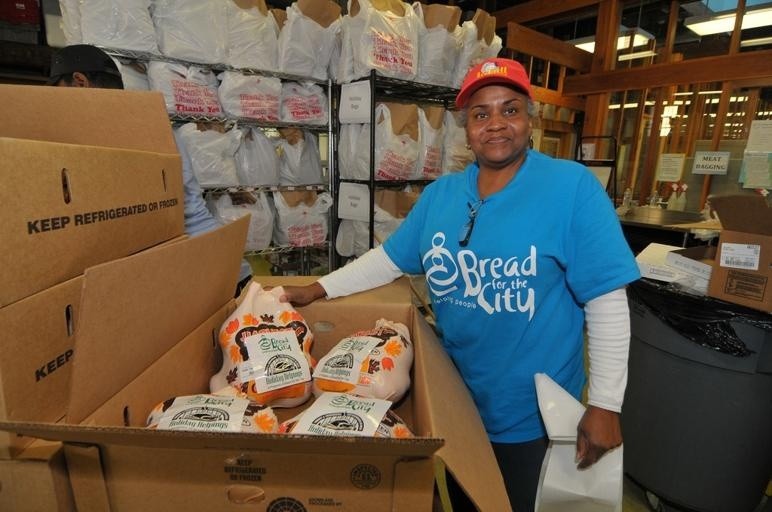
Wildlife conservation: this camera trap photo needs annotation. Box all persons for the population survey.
[261,57,643,512]
[48,43,253,299]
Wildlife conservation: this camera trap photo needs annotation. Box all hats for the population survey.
[48,45,121,87]
[454,57,535,108]
